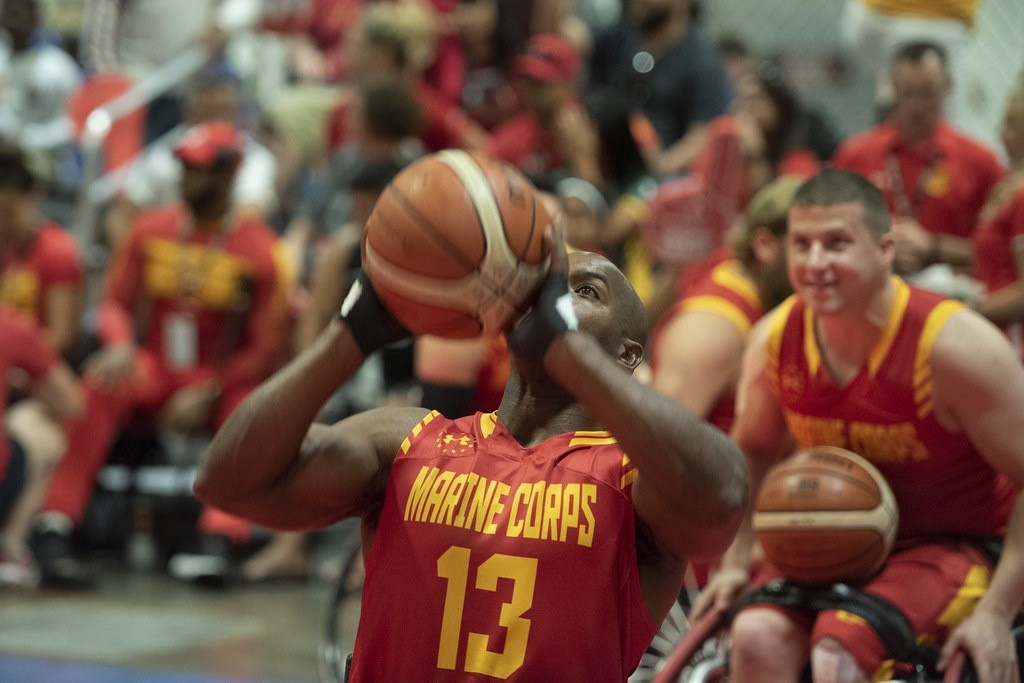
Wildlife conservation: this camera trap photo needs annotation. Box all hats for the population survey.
[513,33,579,78]
[173,120,244,167]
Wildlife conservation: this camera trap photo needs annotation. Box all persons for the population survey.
[192,212,740,683]
[689,171,1024,683]
[0,0,1024,591]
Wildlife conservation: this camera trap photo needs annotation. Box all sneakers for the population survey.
[171,534,232,582]
[33,531,92,589]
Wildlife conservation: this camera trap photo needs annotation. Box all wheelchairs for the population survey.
[625,537,1022,683]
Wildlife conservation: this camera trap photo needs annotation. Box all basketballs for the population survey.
[364,149,559,340]
[750,447,904,587]
